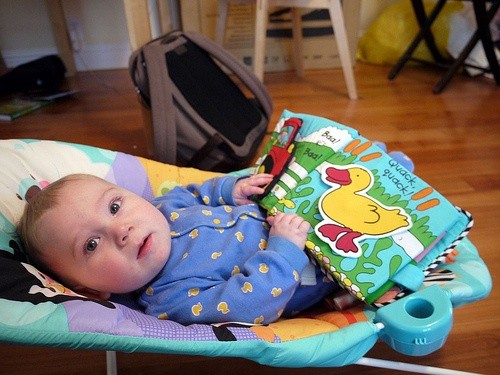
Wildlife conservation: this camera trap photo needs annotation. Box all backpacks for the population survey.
[128,29,273,172]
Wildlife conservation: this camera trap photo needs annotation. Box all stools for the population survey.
[217,1,358,100]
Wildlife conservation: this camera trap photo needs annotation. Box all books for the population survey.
[247,110,474,307]
[0,86,78,120]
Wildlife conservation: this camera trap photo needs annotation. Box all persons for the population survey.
[14,141,416,324]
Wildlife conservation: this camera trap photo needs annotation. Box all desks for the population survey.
[387,0,500,94]
